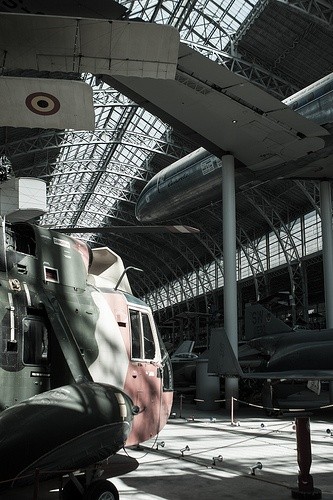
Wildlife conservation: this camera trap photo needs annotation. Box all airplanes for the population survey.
[98,15,333,224]
[0,10,179,132]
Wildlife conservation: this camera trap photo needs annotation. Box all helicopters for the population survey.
[0,175,201,500]
